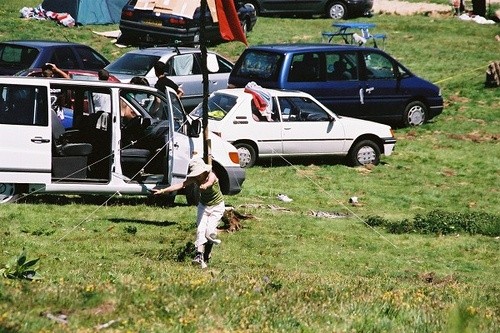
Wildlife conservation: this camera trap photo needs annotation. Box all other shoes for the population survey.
[191,254,204,265]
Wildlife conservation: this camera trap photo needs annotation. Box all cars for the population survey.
[239,0,374,20]
[186,86,399,171]
[4,69,124,119]
[101,46,238,121]
[118,0,258,49]
[0,39,112,79]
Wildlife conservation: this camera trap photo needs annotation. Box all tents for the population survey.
[41,0,129,25]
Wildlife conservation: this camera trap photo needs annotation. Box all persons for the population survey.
[152,157,225,265]
[150,61,184,118]
[92,69,111,113]
[41,63,70,106]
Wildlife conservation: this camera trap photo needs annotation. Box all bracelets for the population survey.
[155,108,159,112]
[161,189,164,193]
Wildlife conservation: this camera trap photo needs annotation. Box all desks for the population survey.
[332,21,375,38]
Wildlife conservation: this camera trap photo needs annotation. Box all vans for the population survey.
[228,42,447,130]
[0,74,247,210]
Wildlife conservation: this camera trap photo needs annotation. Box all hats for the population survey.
[187,154,212,177]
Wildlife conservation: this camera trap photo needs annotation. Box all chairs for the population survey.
[51,109,152,180]
[271,103,280,122]
[292,60,317,82]
[329,61,352,80]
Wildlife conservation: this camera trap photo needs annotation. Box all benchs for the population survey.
[322,32,357,44]
[369,34,387,50]
